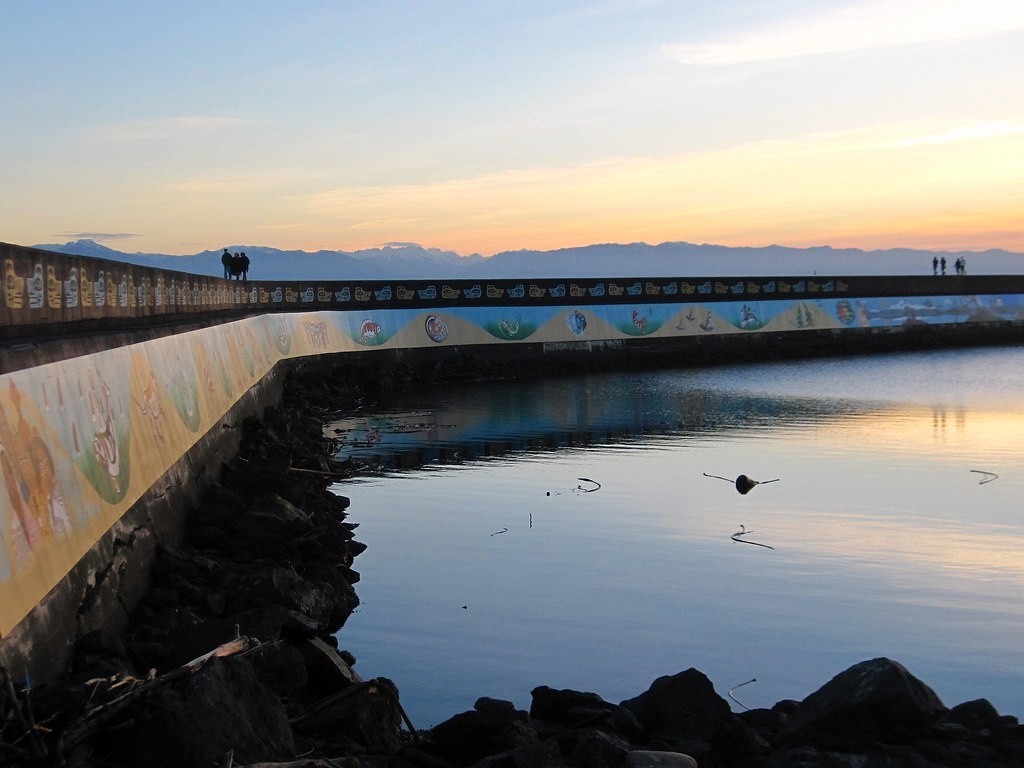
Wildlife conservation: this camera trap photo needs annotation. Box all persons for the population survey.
[960,256,967,275]
[240,252,250,280]
[231,253,241,280]
[222,249,232,279]
[932,257,939,275]
[940,257,946,274]
[954,258,961,275]
